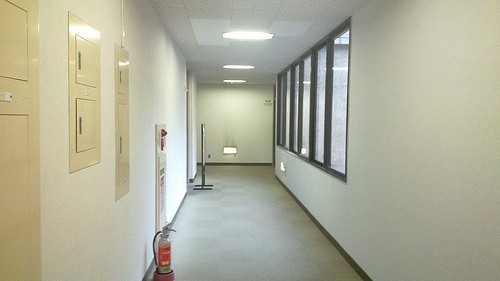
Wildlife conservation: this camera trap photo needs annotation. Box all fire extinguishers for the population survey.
[153,221,177,275]
